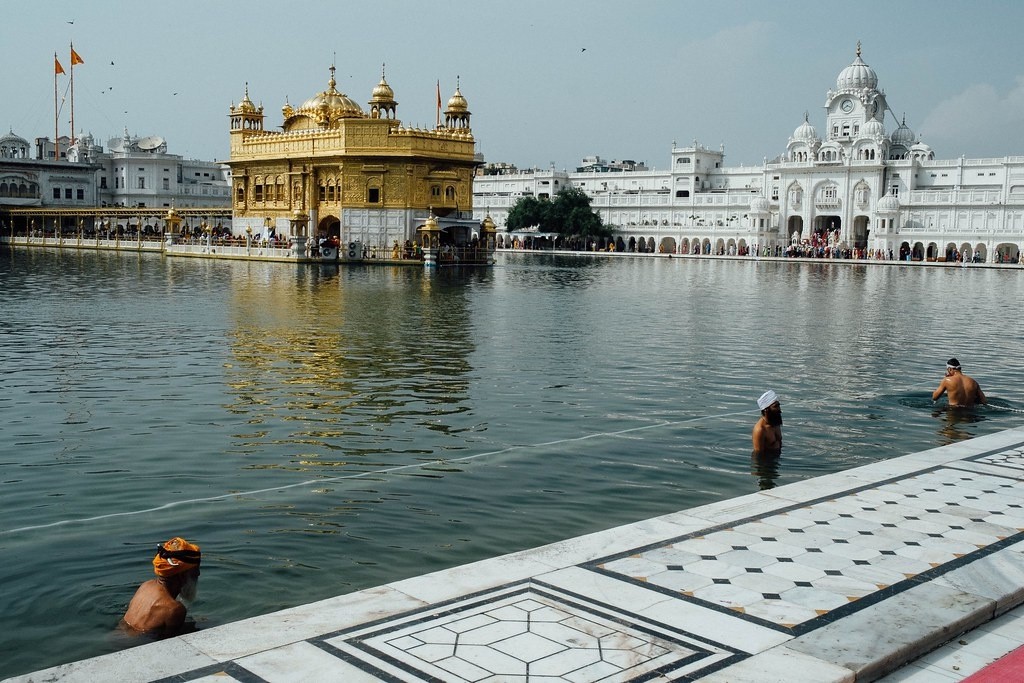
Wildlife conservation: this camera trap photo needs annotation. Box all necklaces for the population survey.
[761,416,770,424]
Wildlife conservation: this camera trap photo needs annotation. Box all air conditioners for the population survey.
[347,240,360,260]
[320,239,337,260]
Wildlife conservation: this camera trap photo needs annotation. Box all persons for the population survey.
[1,218,1023,265]
[113,537,202,640]
[752,390,783,454]
[931,358,987,407]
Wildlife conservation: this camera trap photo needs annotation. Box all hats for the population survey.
[757,390,780,409]
[153,538,201,574]
